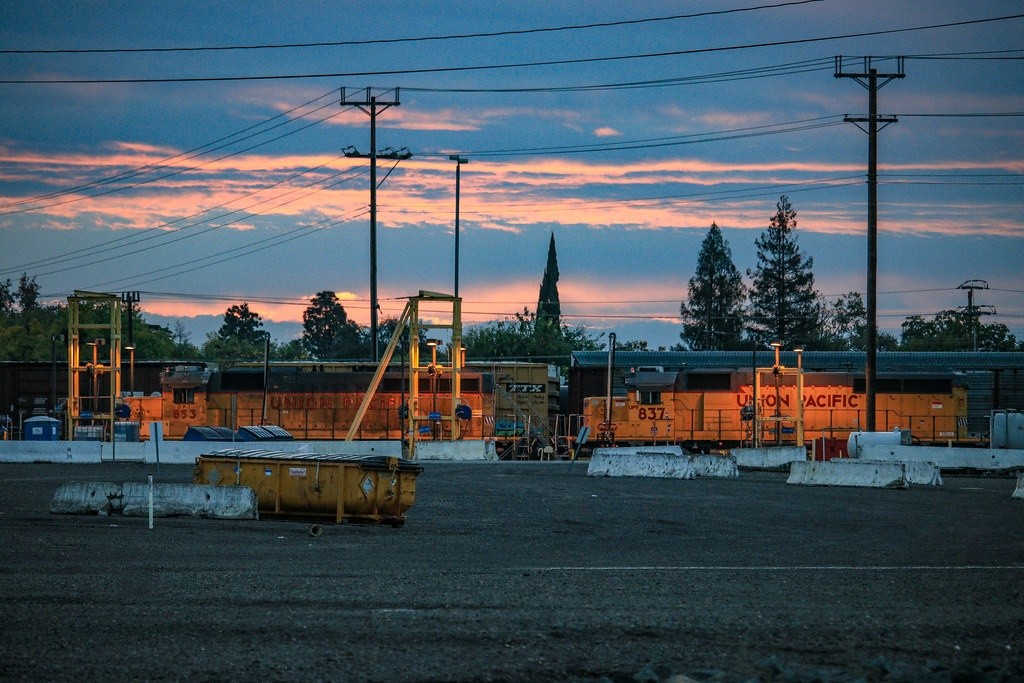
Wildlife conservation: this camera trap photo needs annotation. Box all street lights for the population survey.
[125,343,137,395]
[399,334,412,453]
[49,334,65,418]
[767,340,782,448]
[793,345,805,446]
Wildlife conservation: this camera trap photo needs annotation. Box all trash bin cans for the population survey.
[22,416,60,441]
[74,421,139,442]
